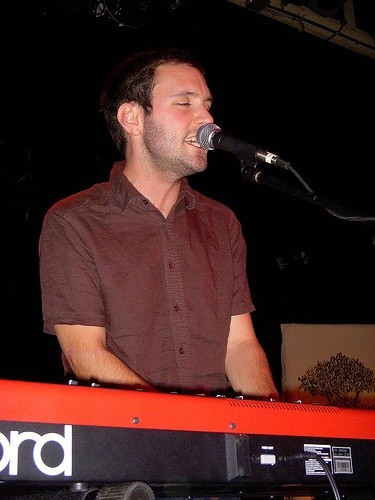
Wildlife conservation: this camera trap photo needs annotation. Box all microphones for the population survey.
[198,124,293,171]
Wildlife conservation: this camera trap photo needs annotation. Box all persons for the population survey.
[37,41,285,403]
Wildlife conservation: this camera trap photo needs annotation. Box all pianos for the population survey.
[0,381,375,493]
